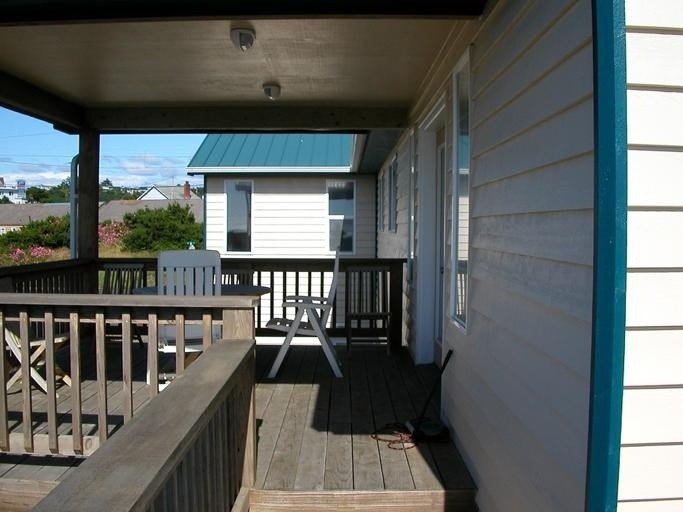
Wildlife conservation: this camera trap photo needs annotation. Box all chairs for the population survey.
[1,273,75,399]
[262,251,346,383]
[158,246,223,378]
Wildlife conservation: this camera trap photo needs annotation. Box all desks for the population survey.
[124,281,265,379]
